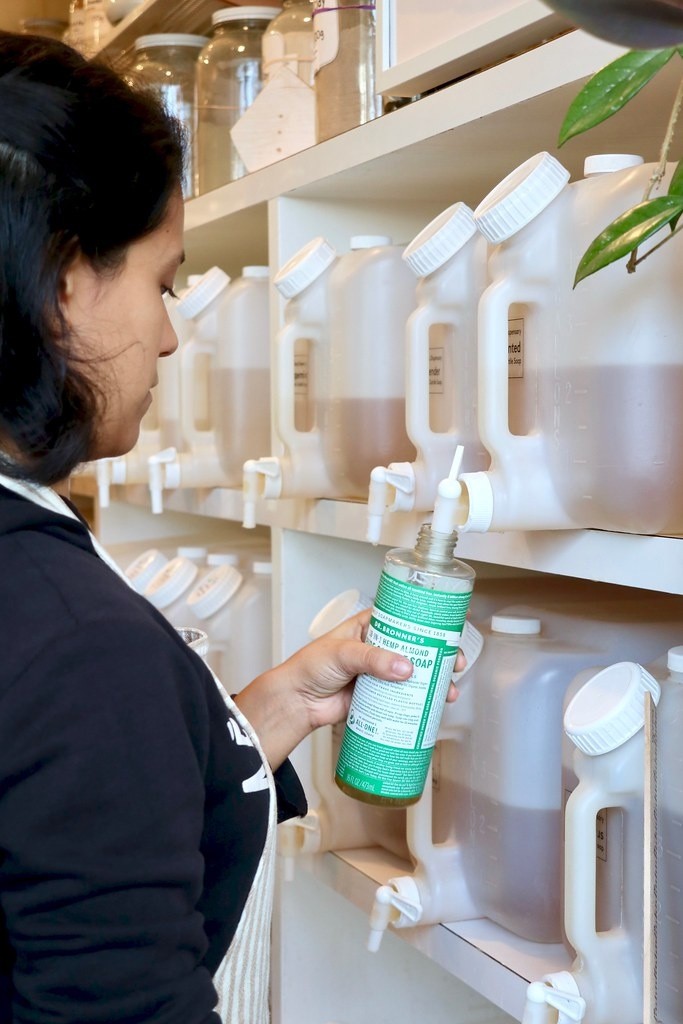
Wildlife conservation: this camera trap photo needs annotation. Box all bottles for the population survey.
[95,149,683,1024]
[336,523,477,810]
[261,0,317,158]
[310,0,386,142]
[192,5,284,196]
[123,32,212,202]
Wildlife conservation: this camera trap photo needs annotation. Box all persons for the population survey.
[0,29,467,1024]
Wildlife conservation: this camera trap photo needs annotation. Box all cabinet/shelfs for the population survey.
[66,0,683,1024]
